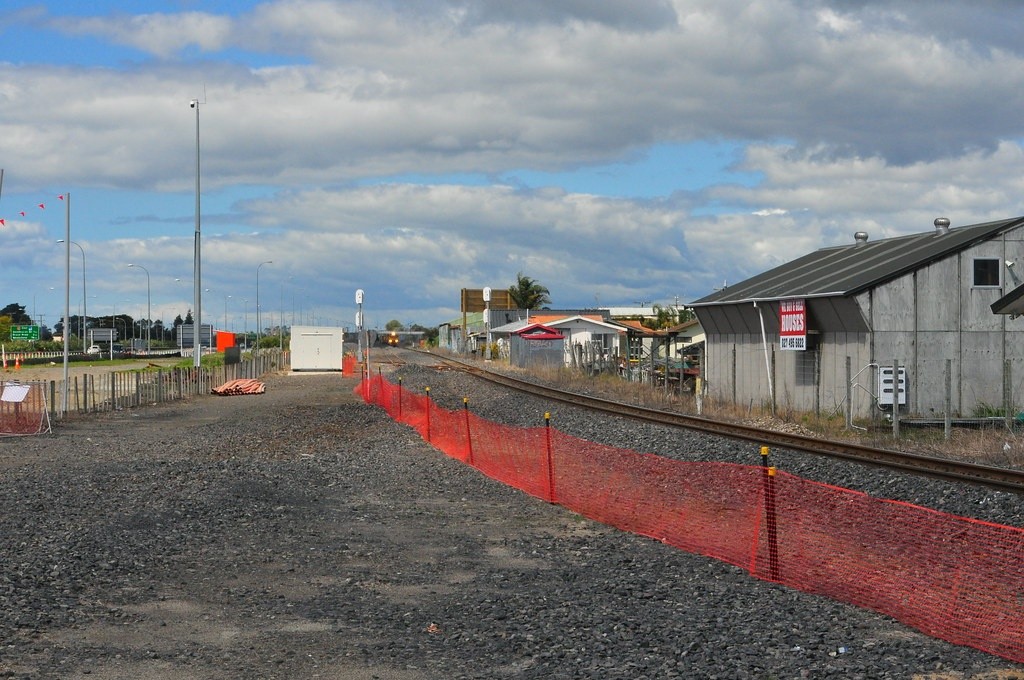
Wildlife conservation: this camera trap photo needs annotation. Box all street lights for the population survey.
[224,295,232,330]
[279,274,296,351]
[256,261,273,355]
[128,264,151,354]
[112,300,130,328]
[77,295,97,347]
[161,307,176,342]
[33,288,54,325]
[56,239,88,355]
[116,317,126,343]
[139,304,156,341]
[15,357,21,371]
[188,99,202,368]
[257,304,263,341]
[242,297,249,355]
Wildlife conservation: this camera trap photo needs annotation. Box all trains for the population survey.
[382,331,399,347]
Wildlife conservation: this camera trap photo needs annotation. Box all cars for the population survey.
[112,344,126,355]
[87,345,101,355]
